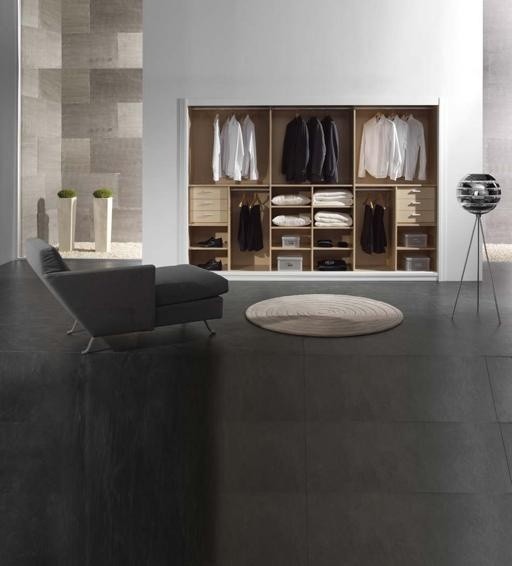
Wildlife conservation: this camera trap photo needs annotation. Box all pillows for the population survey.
[271,193,311,228]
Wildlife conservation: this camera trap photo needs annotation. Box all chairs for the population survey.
[23,234,233,356]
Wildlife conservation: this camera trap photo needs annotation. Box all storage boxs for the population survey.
[275,254,303,272]
[402,232,429,248]
[280,233,301,247]
[403,255,432,272]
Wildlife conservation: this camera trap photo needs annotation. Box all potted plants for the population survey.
[92,189,114,253]
[58,190,78,252]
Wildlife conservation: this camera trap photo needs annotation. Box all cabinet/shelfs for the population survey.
[179,95,447,280]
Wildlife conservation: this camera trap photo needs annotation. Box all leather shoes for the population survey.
[199,238,222,247]
[199,260,222,270]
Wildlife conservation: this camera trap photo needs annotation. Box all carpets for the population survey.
[245,292,409,341]
